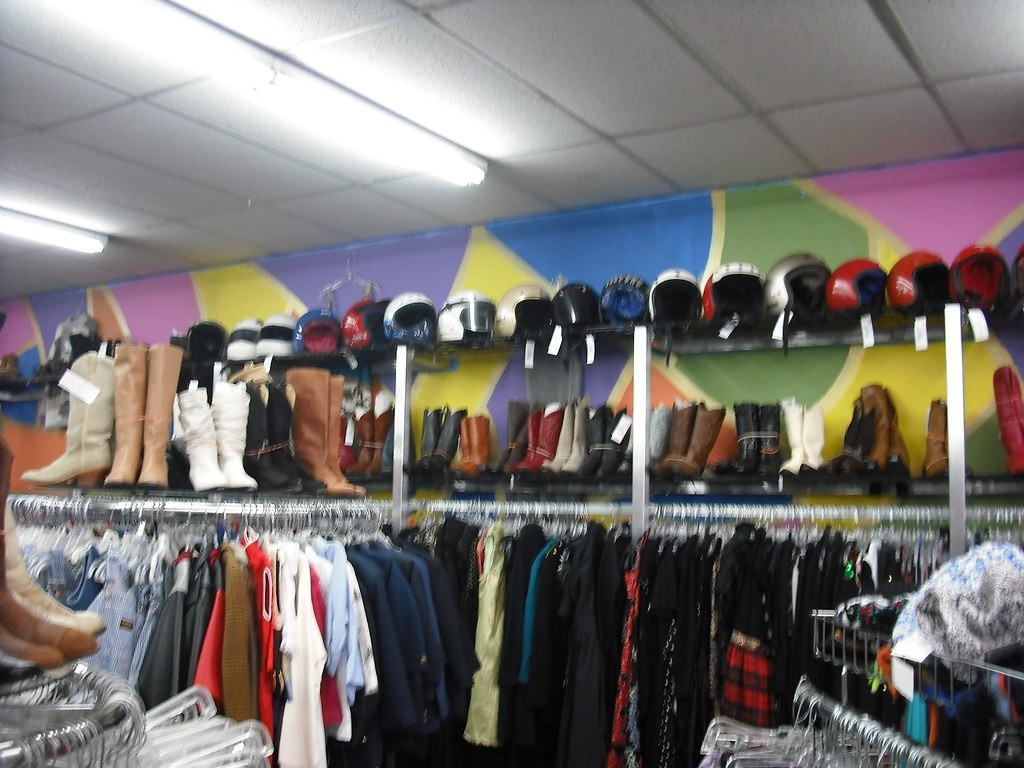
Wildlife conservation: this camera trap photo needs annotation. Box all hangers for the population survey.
[0,495,1024,768]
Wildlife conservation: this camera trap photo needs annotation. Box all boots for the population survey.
[1,436,107,682]
[0,351,20,387]
[22,341,1024,494]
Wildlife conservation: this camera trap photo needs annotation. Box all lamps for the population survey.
[0,206,110,255]
[34,0,490,187]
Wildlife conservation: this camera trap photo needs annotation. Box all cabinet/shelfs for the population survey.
[648,310,948,497]
[958,306,1024,494]
[223,343,396,494]
[407,318,631,496]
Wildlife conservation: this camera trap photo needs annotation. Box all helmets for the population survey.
[51,245,1024,381]
